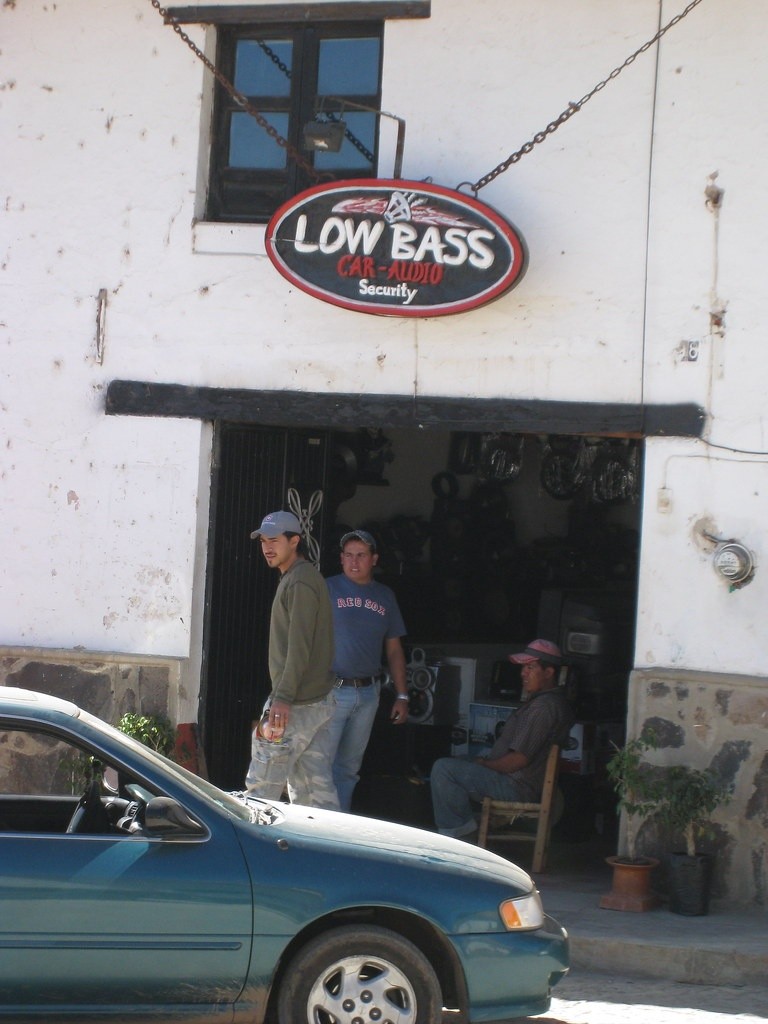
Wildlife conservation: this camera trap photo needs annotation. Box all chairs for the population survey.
[478,744,562,875]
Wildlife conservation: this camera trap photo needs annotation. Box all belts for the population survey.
[333,676,379,687]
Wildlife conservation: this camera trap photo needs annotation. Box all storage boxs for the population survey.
[467,700,596,777]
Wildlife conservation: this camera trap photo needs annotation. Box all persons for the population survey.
[431,638,571,845]
[319,531,409,816]
[246,511,340,813]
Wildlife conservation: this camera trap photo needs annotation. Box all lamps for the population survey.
[302,119,348,154]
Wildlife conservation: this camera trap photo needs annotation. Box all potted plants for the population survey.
[660,761,736,917]
[598,728,682,914]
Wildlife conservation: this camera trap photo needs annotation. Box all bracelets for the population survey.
[396,694,410,702]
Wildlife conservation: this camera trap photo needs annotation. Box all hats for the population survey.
[508,639,562,666]
[250,511,302,539]
[341,529,375,553]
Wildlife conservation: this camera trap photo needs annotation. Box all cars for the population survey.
[0,684,575,1024]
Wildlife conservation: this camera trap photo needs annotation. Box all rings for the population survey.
[275,715,279,717]
